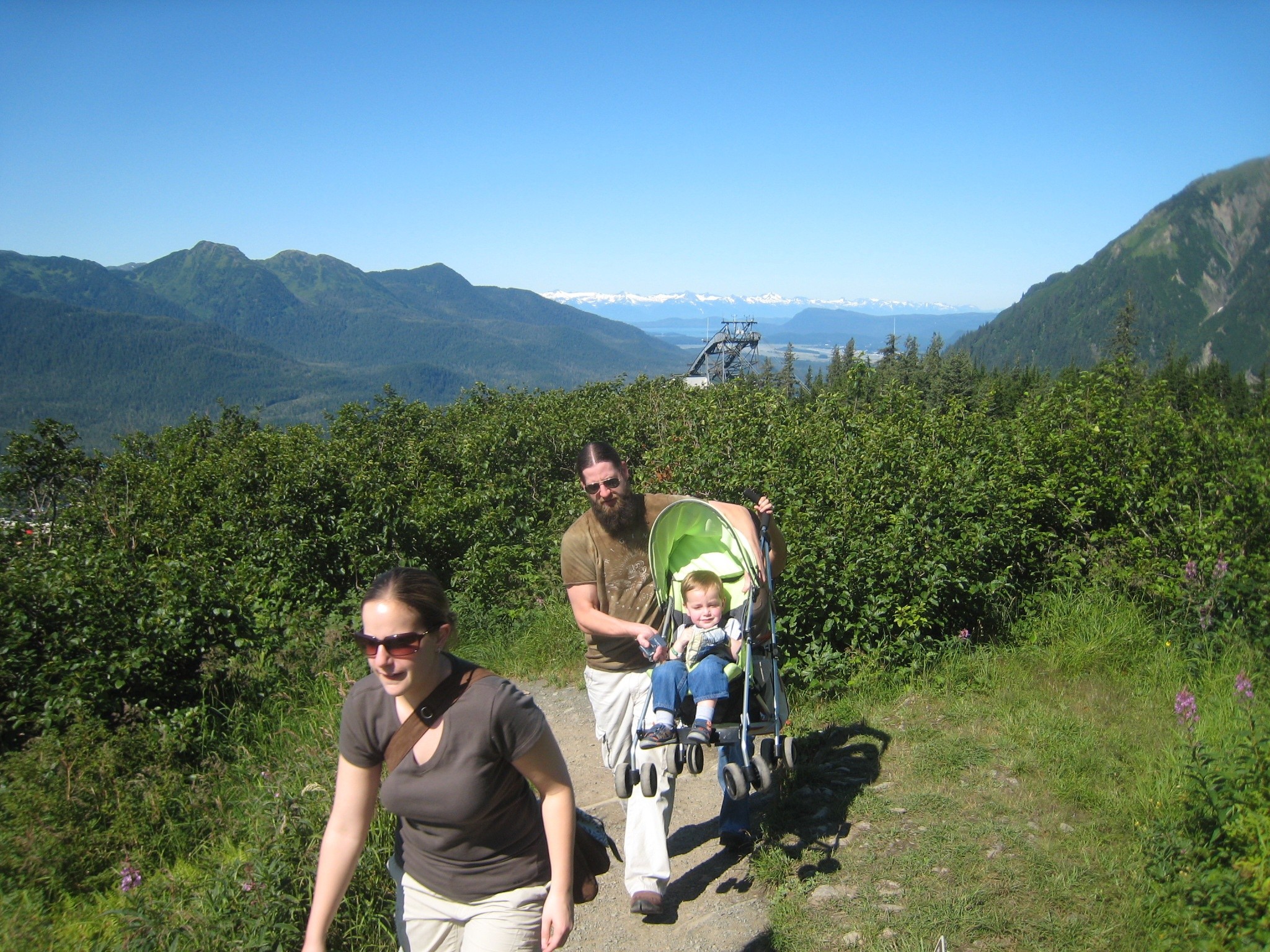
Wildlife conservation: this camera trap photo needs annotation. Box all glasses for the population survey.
[354,624,440,656]
[584,466,621,495]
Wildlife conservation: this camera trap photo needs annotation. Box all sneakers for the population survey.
[640,723,676,750]
[687,719,712,742]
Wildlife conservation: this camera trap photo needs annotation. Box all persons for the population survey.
[560,442,768,914]
[719,496,787,845]
[300,567,577,951]
[639,570,742,749]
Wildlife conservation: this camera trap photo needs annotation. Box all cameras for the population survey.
[638,634,667,658]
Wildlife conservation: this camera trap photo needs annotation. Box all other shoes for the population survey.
[719,831,746,847]
[630,891,664,915]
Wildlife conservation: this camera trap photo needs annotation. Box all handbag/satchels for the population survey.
[751,655,789,736]
[572,807,622,904]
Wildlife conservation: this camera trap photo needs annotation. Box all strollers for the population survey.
[612,485,799,800]
